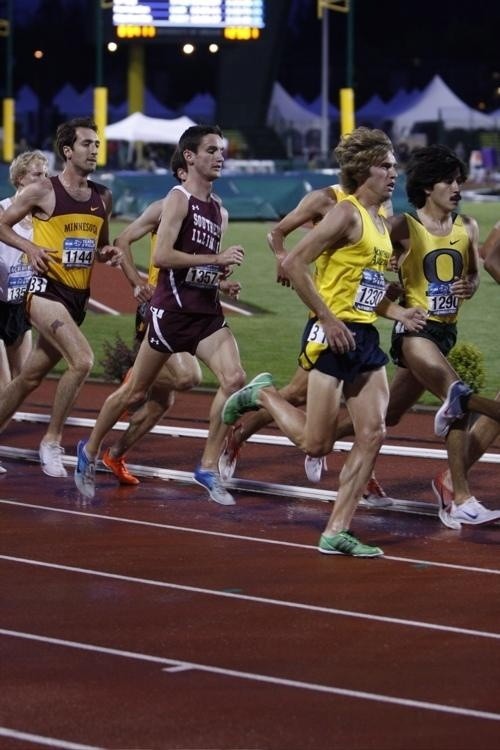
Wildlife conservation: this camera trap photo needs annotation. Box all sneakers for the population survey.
[216,426,243,480]
[362,468,394,508]
[193,462,238,507]
[433,378,473,438]
[37,438,68,479]
[449,496,500,526]
[74,438,96,501]
[220,370,275,426]
[101,447,140,486]
[317,529,382,559]
[320,455,330,472]
[303,453,326,484]
[431,469,463,531]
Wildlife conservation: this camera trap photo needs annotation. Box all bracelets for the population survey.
[468,279,477,300]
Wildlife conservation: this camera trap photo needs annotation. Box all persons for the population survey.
[221,127,428,559]
[432,219,500,531]
[216,124,394,507]
[0,119,124,479]
[0,148,48,393]
[75,125,236,508]
[304,145,499,525]
[100,146,241,485]
[431,240,500,437]
